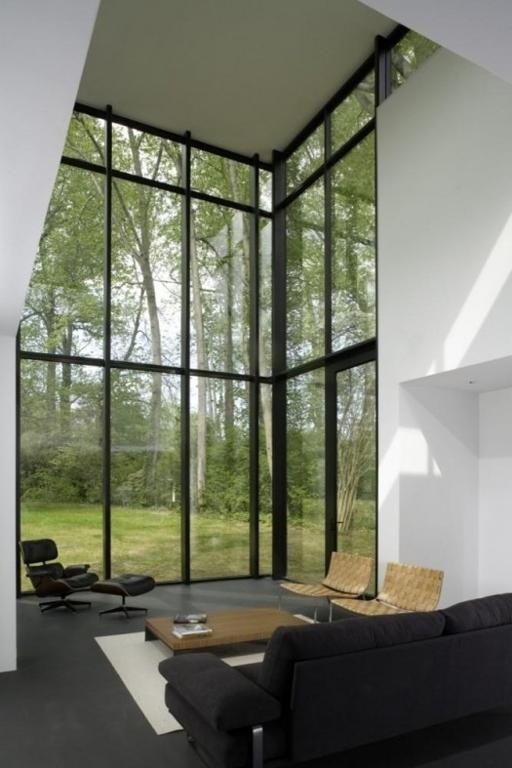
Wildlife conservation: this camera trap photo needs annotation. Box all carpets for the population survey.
[94,613,318,735]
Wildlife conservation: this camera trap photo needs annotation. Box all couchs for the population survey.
[159,592,512,768]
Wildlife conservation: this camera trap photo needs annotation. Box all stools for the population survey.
[91,573,155,618]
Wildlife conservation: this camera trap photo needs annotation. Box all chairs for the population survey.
[278,551,374,623]
[19,538,98,612]
[330,562,445,623]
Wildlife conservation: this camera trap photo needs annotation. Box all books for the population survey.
[172,612,211,640]
